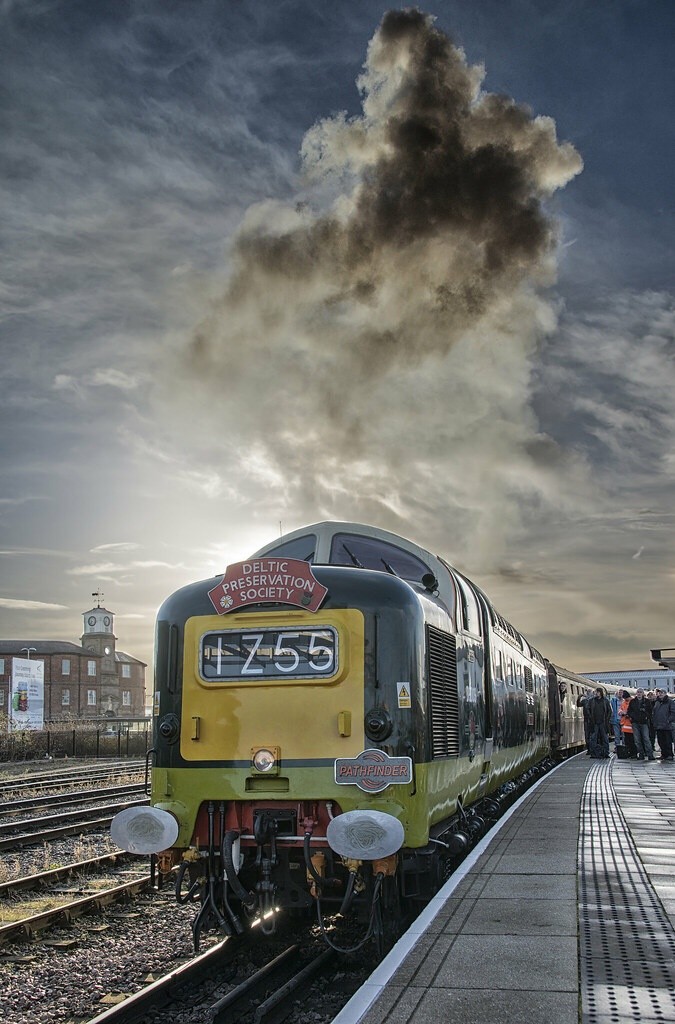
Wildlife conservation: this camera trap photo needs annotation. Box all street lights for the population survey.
[21,647,36,659]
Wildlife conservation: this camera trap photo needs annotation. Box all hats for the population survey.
[657,688,666,693]
[622,690,630,699]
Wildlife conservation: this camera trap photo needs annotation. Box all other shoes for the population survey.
[649,756,656,760]
[637,756,644,760]
[586,752,590,755]
[660,755,668,759]
[605,755,610,758]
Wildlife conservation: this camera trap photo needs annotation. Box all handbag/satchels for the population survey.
[617,743,628,759]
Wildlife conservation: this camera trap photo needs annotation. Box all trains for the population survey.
[110,520,675,951]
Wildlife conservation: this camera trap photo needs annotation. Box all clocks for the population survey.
[103,616,110,627]
[88,616,96,626]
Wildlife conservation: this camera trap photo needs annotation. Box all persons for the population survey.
[576,687,675,760]
[559,682,567,712]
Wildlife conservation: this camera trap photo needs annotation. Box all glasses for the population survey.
[658,691,665,695]
